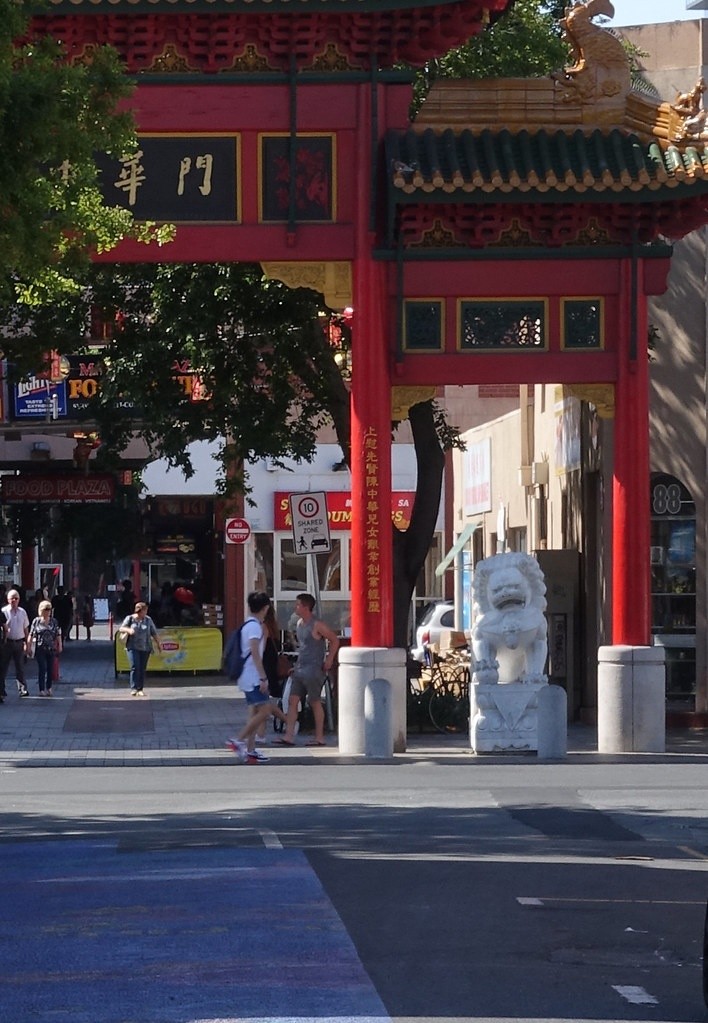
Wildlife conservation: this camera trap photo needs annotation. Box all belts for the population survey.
[8,639,24,642]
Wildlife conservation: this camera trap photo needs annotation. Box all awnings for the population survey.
[435,521,478,576]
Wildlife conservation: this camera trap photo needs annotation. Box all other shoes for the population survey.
[131,690,137,695]
[137,691,145,696]
[245,734,266,745]
[293,721,299,736]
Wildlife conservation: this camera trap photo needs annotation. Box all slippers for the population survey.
[271,738,295,746]
[304,740,326,747]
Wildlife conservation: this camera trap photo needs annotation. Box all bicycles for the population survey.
[406,661,472,734]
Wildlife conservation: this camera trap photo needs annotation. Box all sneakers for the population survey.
[243,748,271,762]
[225,736,248,763]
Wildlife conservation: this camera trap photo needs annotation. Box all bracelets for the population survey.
[259,676,268,682]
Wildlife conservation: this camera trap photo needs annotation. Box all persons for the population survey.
[119,602,162,696]
[0,589,30,696]
[244,602,300,743]
[27,600,62,696]
[226,591,274,762]
[0,580,202,643]
[0,608,9,703]
[270,593,339,746]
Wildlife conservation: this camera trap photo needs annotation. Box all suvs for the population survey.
[415,601,454,663]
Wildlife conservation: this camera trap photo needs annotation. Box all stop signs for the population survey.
[226,518,251,544]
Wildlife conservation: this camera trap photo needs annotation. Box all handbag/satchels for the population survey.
[23,638,36,660]
[276,653,290,677]
[119,615,132,644]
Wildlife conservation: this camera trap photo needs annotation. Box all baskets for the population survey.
[405,659,423,678]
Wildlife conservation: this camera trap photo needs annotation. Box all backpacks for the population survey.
[218,619,265,683]
[35,589,44,602]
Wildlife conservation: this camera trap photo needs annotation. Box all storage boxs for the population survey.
[201,603,221,612]
[204,619,224,627]
[204,611,224,619]
